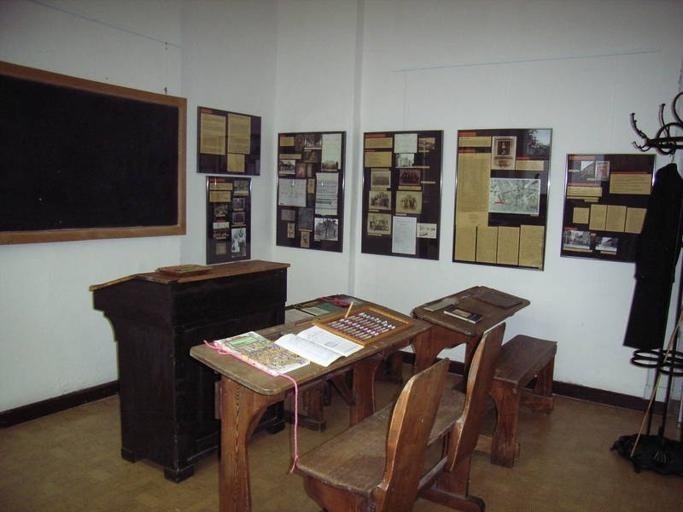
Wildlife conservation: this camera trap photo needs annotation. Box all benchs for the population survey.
[293,322,506,511]
[473,335,556,468]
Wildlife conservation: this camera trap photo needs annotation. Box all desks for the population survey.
[411,286,528,367]
[189,303,430,511]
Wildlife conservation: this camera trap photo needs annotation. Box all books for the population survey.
[214,331,310,377]
[443,306,484,324]
[274,325,365,368]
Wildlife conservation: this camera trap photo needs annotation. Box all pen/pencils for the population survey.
[327,310,404,341]
[344,302,353,320]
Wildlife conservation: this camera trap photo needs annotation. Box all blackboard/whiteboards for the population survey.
[0,61,186,245]
